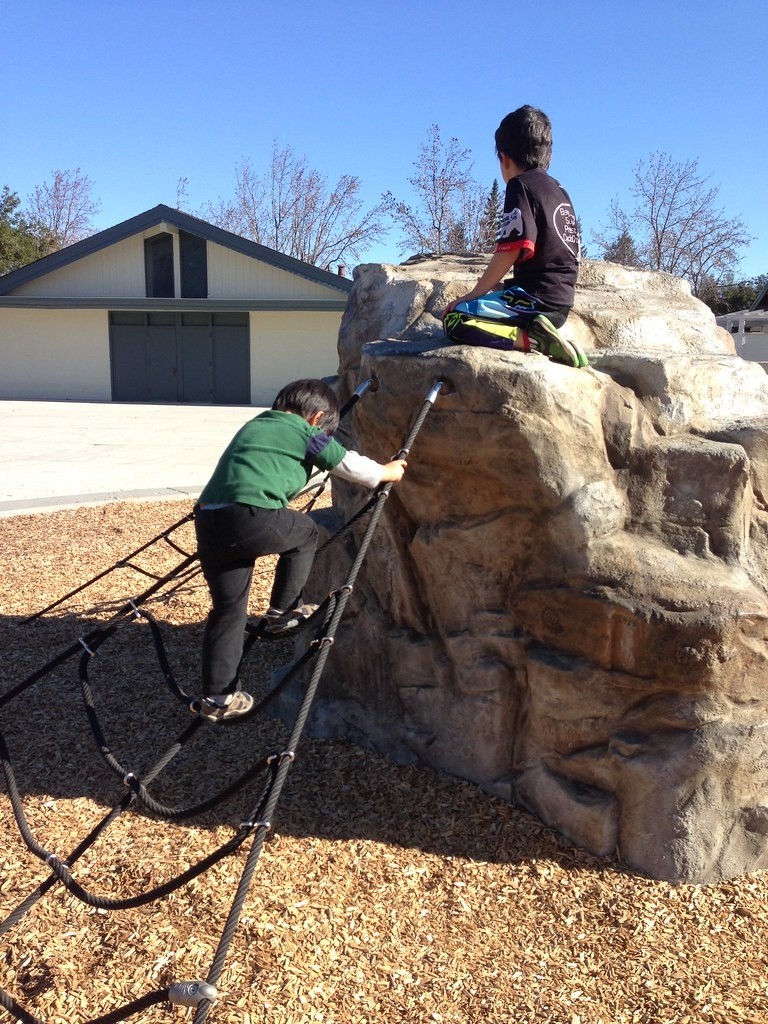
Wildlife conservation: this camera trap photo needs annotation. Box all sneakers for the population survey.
[524,313,587,367]
[201,691,254,722]
[267,603,320,634]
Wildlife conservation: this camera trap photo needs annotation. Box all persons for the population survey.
[438,99,597,371]
[188,376,413,724]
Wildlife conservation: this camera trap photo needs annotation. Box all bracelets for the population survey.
[472,289,478,299]
